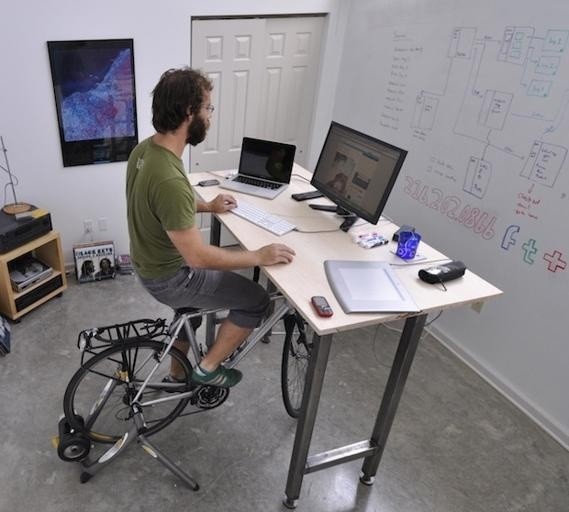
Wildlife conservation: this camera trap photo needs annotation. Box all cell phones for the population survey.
[311,296,333,317]
[199,179,219,187]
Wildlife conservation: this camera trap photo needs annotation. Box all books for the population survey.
[118,255,136,275]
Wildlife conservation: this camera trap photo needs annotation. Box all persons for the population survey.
[123,66,297,396]
[78,259,94,281]
[96,258,113,279]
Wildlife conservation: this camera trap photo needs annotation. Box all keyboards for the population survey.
[228,199,296,237]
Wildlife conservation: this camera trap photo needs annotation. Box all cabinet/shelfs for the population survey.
[0,229,68,323]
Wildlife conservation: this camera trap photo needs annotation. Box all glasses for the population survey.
[200,103,214,114]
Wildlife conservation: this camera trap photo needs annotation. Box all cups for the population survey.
[395,231,422,259]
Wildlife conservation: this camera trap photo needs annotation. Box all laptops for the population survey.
[218,137,296,200]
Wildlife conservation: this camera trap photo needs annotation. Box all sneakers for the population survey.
[191,361,242,389]
[165,375,186,393]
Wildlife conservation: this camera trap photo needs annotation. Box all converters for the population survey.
[392,224,416,242]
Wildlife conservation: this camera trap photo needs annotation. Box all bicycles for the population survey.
[63,293,315,445]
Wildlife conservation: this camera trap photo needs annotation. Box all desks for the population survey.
[185,160,505,508]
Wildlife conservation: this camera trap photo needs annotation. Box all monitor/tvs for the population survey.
[309,121,409,232]
